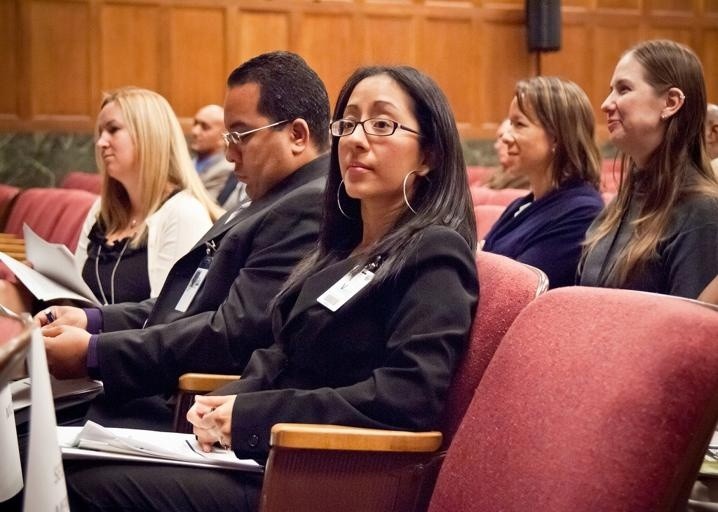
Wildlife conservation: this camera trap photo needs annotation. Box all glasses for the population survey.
[329,117,424,137]
[219,116,305,146]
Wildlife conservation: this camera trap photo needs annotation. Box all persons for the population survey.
[576,39,718,307]
[0,84,228,350]
[480,76,605,294]
[703,101,718,181]
[474,118,533,192]
[188,104,248,212]
[28,49,337,434]
[6,63,481,512]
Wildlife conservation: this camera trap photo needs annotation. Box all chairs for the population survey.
[178,250,550,512]
[427,283,718,512]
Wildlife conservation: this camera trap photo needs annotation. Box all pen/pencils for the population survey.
[44,308,54,323]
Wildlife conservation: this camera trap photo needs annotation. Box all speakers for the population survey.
[524,0,563,52]
